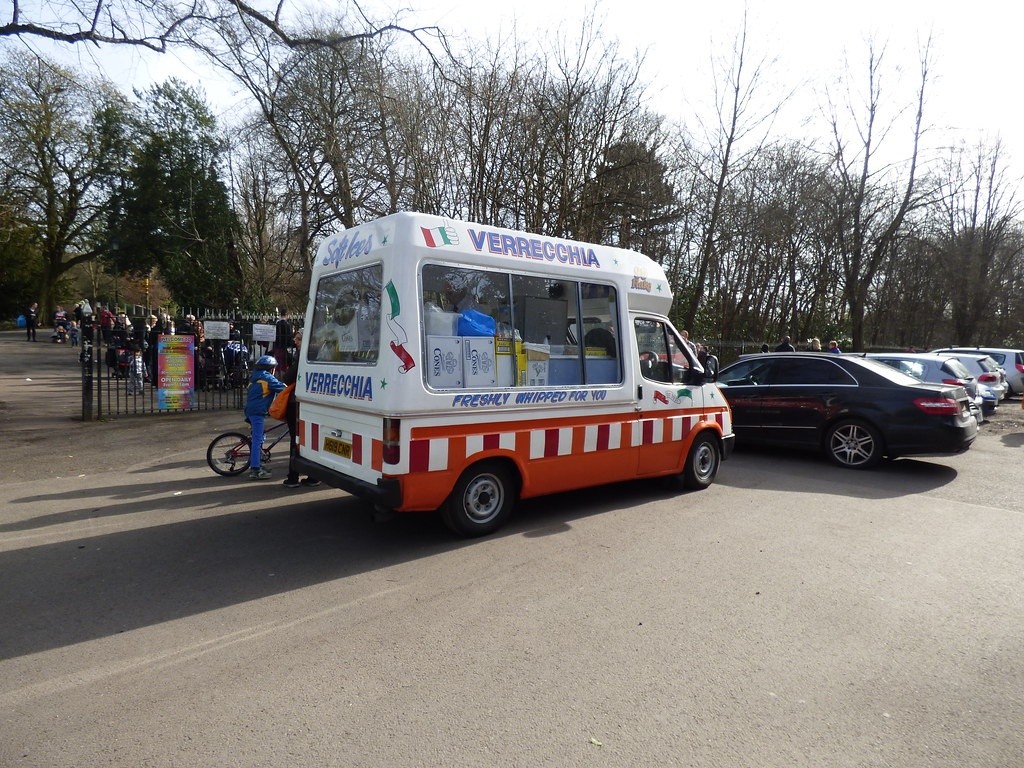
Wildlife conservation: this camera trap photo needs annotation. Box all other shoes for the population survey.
[301,476,322,487]
[249,469,272,478]
[139,390,145,395]
[283,478,299,488]
[128,393,132,395]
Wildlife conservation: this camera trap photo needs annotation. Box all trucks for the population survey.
[291,210,735,527]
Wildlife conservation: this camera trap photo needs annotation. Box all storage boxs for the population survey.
[423,312,618,385]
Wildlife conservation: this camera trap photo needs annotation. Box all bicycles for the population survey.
[206,415,291,478]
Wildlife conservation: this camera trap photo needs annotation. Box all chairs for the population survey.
[583,327,616,359]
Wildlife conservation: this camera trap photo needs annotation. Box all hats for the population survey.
[297,328,304,336]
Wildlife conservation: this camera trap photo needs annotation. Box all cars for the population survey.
[712,352,980,470]
[853,353,1009,423]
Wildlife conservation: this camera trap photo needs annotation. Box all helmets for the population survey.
[255,356,278,365]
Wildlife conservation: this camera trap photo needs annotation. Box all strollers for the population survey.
[223,338,250,388]
[111,337,132,380]
[52,318,68,344]
[202,343,232,392]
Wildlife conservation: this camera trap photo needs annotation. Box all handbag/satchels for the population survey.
[269,383,296,420]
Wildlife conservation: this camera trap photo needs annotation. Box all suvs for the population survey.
[930,347,1024,399]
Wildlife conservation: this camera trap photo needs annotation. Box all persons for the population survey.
[760,335,842,354]
[907,346,927,353]
[27,298,304,396]
[673,330,710,366]
[245,356,319,489]
